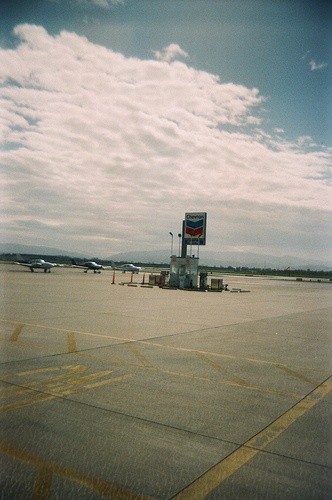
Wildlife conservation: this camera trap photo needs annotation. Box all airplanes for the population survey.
[115,264,141,274]
[17,260,58,273]
[79,262,104,274]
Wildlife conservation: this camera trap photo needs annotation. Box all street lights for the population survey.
[178,233,181,257]
[198,233,203,258]
[170,232,173,256]
